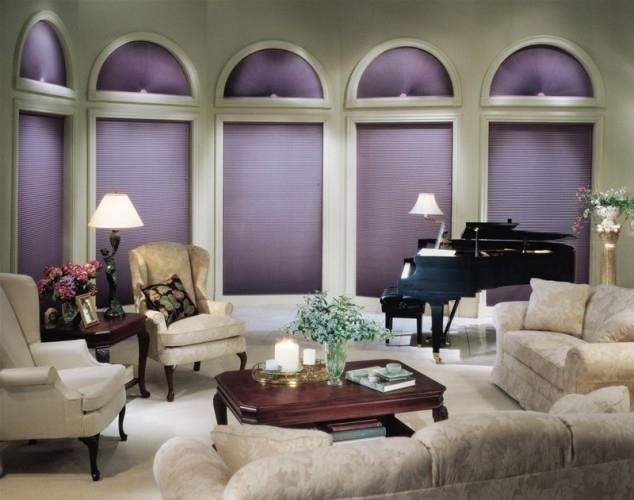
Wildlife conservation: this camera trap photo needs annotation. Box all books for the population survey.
[326,419,386,444]
[345,365,416,393]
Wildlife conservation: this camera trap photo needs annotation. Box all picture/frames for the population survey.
[75,293,101,329]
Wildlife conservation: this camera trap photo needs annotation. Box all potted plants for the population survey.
[279,288,393,386]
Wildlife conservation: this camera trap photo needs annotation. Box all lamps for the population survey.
[87,192,145,319]
[407,191,446,249]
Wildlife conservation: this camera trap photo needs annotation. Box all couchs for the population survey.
[152,385,634,498]
[488,285,634,412]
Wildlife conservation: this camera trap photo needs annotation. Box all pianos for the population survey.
[395,218,576,362]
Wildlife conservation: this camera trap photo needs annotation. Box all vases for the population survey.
[61,304,79,319]
[599,233,618,286]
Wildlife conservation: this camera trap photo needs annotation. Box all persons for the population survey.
[81,300,95,322]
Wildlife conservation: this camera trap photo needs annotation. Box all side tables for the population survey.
[41,312,150,398]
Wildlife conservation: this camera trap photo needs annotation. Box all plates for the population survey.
[375,368,413,381]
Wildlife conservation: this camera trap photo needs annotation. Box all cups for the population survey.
[386,362,401,374]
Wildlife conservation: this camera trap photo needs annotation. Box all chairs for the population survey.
[0,273,129,481]
[129,241,248,402]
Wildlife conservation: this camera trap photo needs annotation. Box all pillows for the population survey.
[143,274,198,323]
[592,309,634,342]
[211,425,334,471]
[523,277,596,338]
[548,383,628,413]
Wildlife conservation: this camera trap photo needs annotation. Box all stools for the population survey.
[382,286,425,347]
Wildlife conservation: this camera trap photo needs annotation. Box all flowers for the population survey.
[37,259,106,303]
[570,184,634,236]
